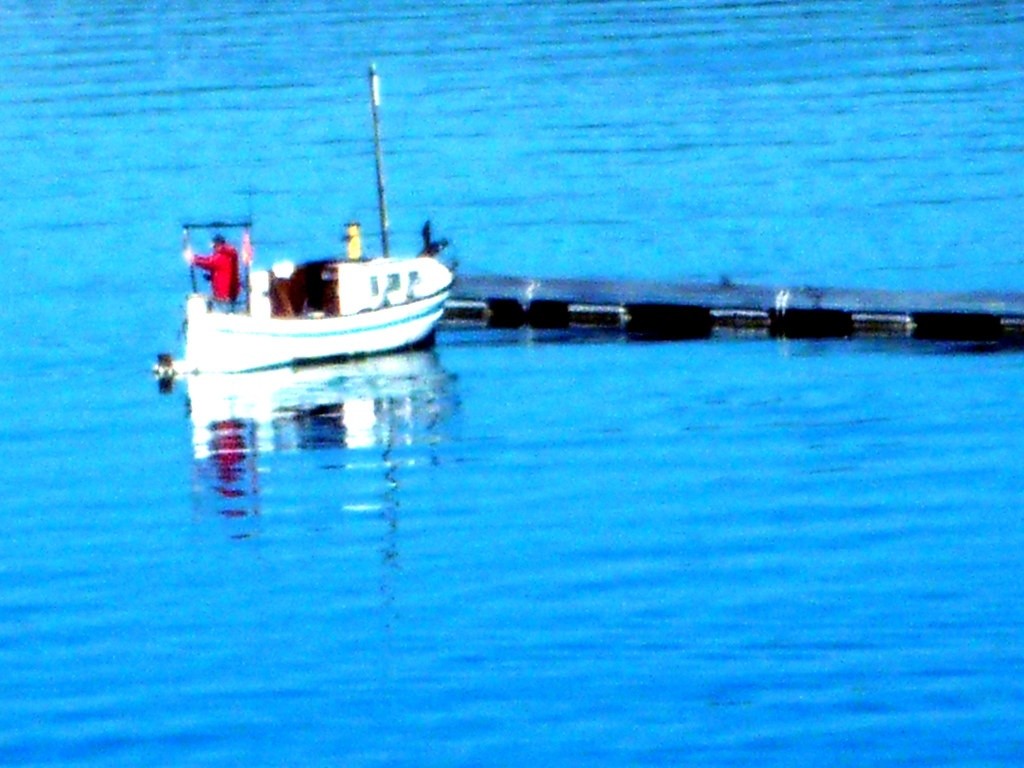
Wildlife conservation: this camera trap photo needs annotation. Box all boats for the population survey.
[156,69,458,379]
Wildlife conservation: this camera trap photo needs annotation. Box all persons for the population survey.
[185,233,240,310]
